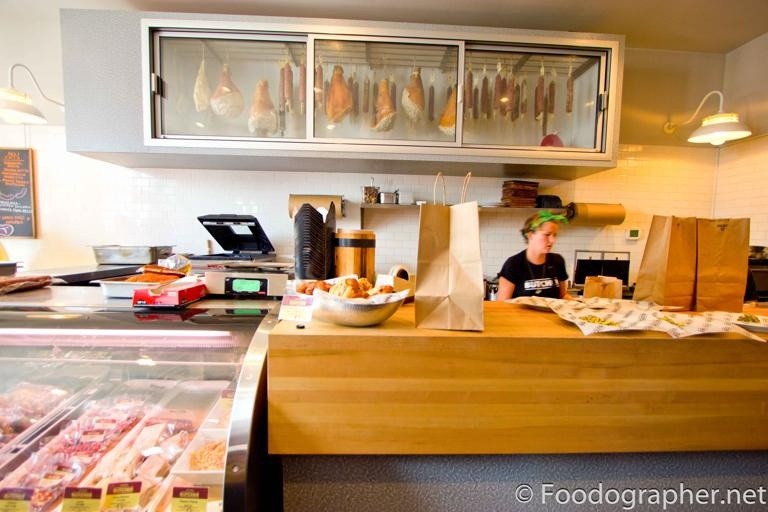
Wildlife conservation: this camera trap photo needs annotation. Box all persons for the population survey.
[495,209,577,301]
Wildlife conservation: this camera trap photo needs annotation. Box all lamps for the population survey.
[1,62,64,127]
[663,90,753,147]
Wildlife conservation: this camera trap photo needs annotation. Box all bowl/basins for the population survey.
[311,296,407,327]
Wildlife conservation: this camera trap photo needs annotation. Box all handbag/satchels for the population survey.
[412,171,486,332]
[583,275,622,300]
[697,216,751,312]
[633,215,697,313]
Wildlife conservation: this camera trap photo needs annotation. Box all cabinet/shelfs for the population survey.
[140,18,617,164]
[0,302,277,512]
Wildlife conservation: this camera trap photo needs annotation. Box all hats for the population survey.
[521,211,568,237]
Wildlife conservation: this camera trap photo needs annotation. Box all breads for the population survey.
[297,278,393,298]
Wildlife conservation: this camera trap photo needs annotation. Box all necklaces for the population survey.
[524,249,547,295]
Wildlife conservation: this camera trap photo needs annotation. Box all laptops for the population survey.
[189,213,276,261]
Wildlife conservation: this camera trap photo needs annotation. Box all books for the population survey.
[501,179,540,208]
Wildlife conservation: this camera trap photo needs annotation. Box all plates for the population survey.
[731,315,768,333]
